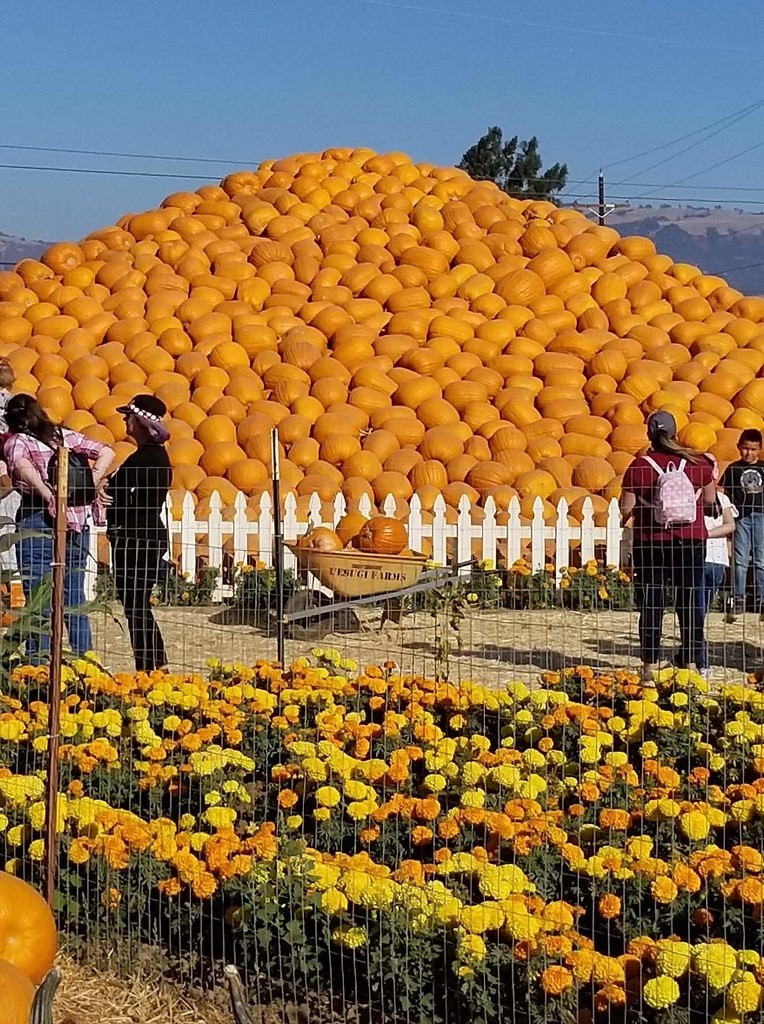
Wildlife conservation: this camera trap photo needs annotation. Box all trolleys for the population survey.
[280,538,501,643]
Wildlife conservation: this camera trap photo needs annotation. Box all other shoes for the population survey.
[699,668,712,680]
[730,600,745,617]
[638,672,656,688]
[146,667,170,676]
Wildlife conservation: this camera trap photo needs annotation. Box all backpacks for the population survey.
[19,423,95,507]
[640,454,702,529]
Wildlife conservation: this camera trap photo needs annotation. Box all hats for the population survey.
[704,452,719,480]
[648,411,675,439]
[116,394,168,423]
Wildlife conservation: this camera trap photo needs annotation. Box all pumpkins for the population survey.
[0,870,58,982]
[0,958,36,1024]
[0,146,764,556]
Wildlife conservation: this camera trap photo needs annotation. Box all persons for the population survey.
[0,357,115,670]
[619,409,716,689]
[699,453,740,676]
[717,428,764,622]
[96,393,173,677]
